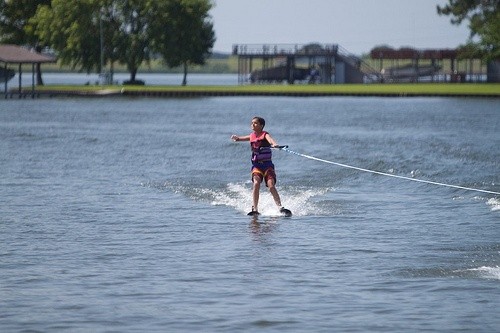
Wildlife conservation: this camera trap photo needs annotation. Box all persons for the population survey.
[231,116,291,217]
